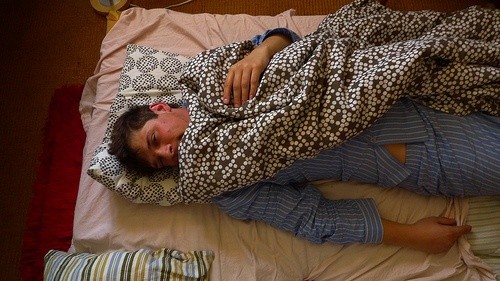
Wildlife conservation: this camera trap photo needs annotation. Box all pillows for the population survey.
[42,249,214,281]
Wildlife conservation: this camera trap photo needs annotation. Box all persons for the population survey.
[106,28,500,254]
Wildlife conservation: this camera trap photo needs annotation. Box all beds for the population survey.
[67,8,500,281]
